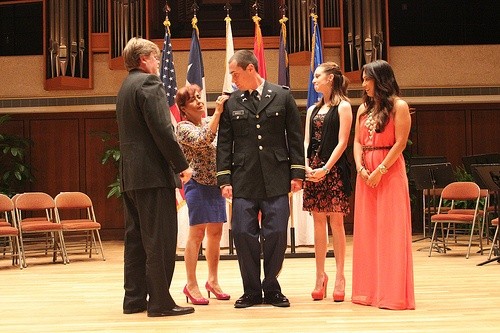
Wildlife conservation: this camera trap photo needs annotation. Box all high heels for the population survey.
[333,278,345,301]
[183,284,209,305]
[205,282,230,300]
[312,272,328,299]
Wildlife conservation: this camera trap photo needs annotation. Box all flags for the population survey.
[278,24,290,86]
[186,25,208,118]
[253,24,267,80]
[159,21,186,210]
[307,21,324,107]
[222,24,238,93]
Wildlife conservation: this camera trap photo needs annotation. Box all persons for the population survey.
[176,84,230,305]
[116,38,194,317]
[216,50,305,308]
[351,60,416,310]
[303,61,352,300]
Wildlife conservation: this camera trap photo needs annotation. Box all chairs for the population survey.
[0,192,105,268]
[423,181,500,260]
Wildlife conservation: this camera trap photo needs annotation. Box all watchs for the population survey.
[378,164,387,174]
[322,166,329,174]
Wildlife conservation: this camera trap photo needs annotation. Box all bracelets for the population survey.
[358,167,364,173]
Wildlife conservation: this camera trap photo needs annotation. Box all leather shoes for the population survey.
[148,304,194,317]
[264,294,290,306]
[123,301,149,314]
[234,295,262,307]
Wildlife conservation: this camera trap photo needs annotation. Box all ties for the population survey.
[251,90,260,111]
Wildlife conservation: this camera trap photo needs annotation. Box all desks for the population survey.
[175,181,329,256]
